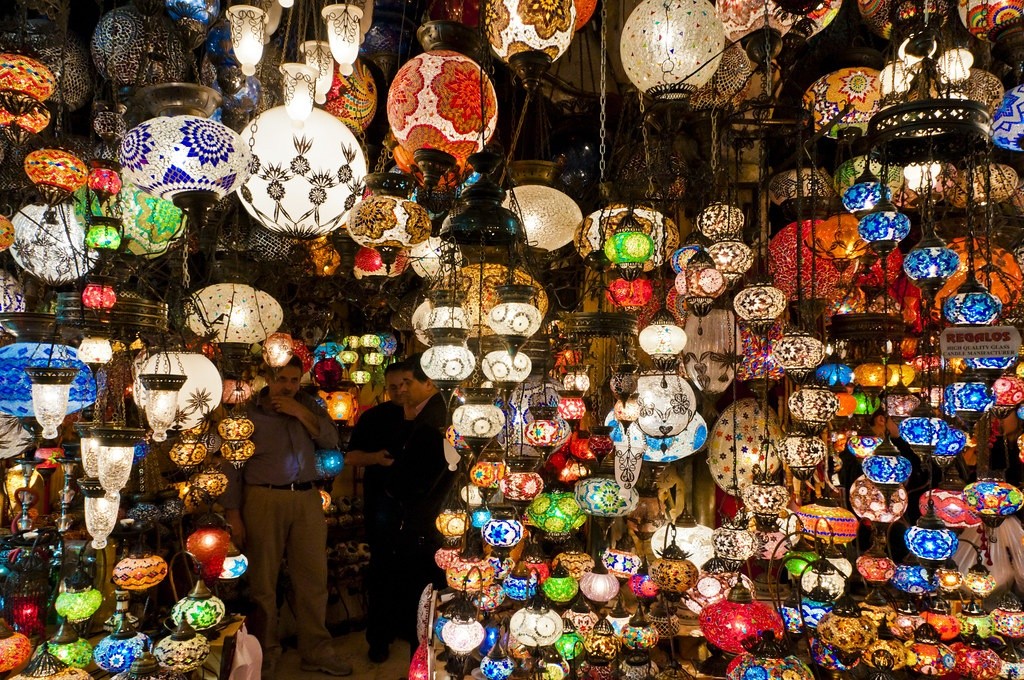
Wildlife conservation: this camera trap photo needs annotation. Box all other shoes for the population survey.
[368,629,390,664]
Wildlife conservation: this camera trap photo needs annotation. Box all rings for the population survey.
[278,404,280,408]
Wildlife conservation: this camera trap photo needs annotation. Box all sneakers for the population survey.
[298,654,354,675]
[261,645,281,678]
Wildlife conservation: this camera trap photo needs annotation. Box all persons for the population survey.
[344,362,404,665]
[963,402,1024,527]
[220,352,354,679]
[844,408,926,591]
[399,352,455,664]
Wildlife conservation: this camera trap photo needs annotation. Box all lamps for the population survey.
[0,1,1023,680]
